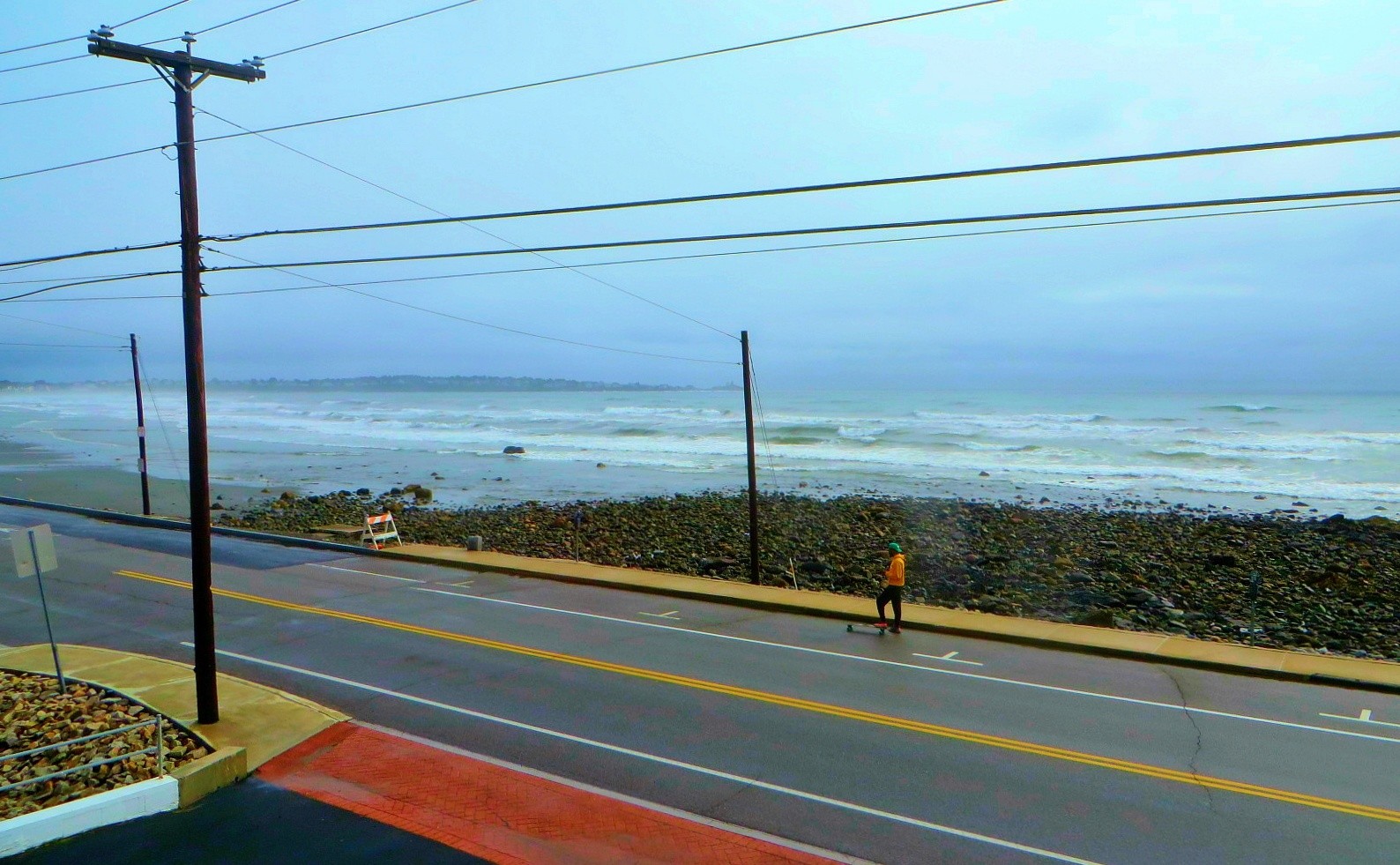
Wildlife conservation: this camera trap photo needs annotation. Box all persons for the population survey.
[872,542,906,634]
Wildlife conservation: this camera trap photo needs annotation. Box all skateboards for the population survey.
[847,623,886,634]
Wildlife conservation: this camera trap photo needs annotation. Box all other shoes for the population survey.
[874,622,888,628]
[889,626,902,633]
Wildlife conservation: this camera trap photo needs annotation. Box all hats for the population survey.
[888,542,903,552]
